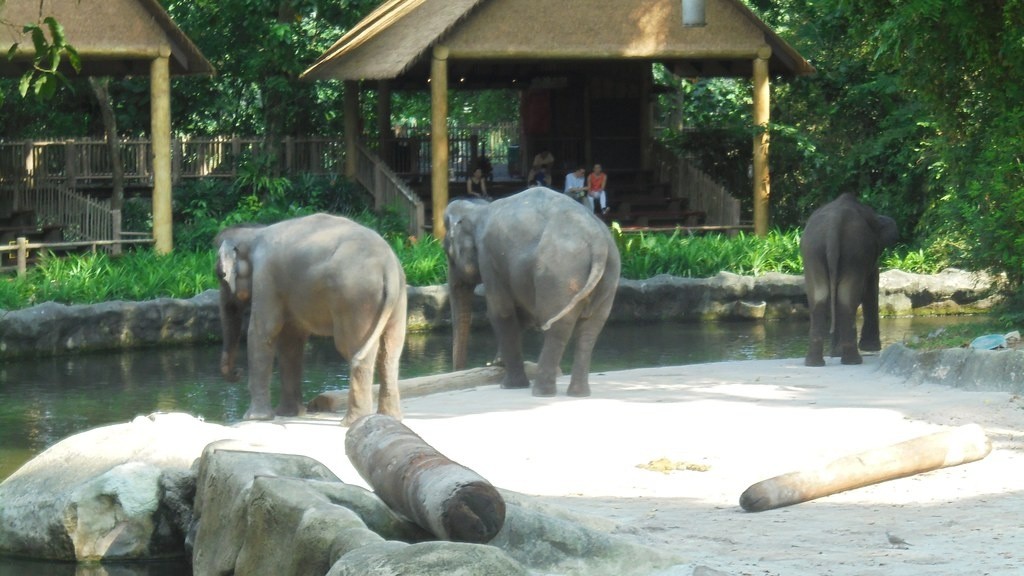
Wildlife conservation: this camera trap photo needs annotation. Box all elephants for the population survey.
[213,211,408,427]
[798,192,899,367]
[442,186,622,398]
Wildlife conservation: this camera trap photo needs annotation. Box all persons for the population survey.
[586,161,611,217]
[507,159,521,180]
[527,145,555,191]
[563,164,591,211]
[464,166,494,203]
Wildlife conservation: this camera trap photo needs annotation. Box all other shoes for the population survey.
[601,207,611,215]
